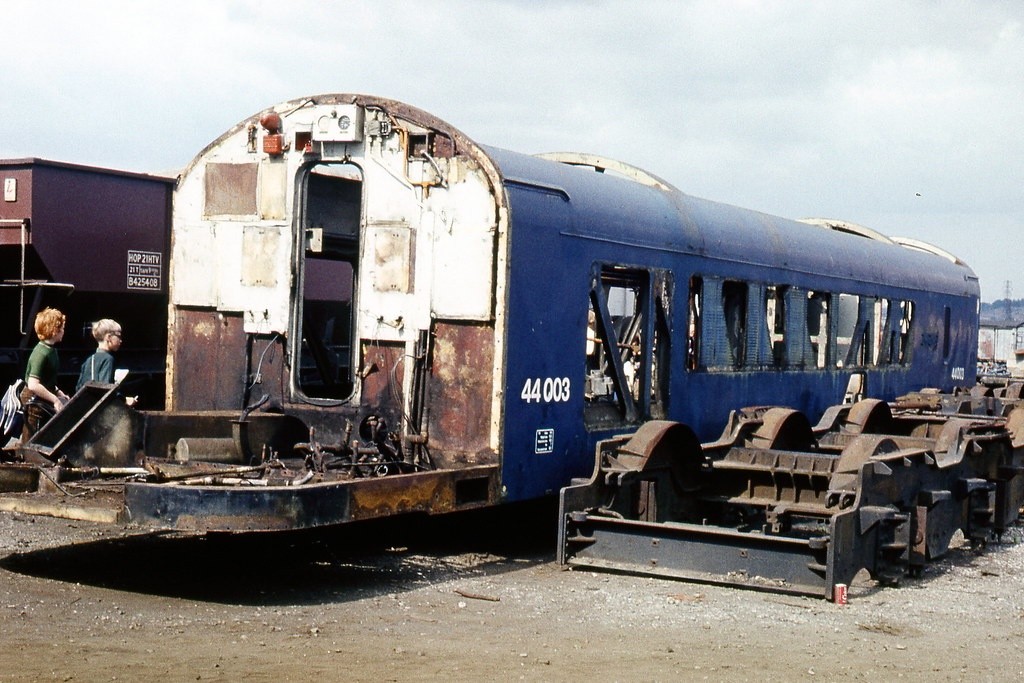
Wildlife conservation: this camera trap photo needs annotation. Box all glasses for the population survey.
[114,334,122,339]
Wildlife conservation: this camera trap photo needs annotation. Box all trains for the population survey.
[114,92,982,533]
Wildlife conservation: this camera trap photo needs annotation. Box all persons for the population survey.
[77,317,137,409]
[20,308,70,444]
